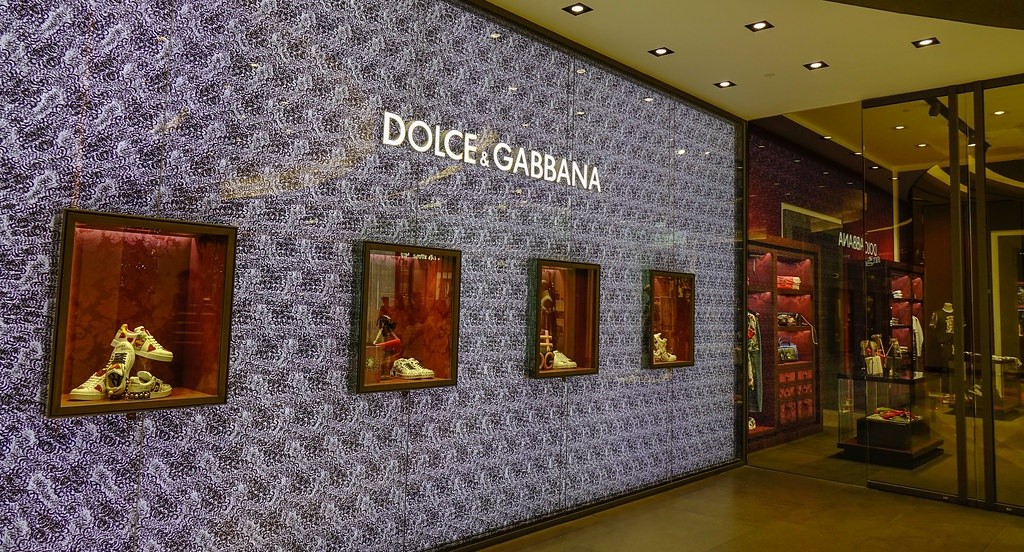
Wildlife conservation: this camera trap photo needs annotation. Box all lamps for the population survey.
[967,133,976,147]
[929,103,941,117]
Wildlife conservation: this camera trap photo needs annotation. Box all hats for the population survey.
[942,302,954,313]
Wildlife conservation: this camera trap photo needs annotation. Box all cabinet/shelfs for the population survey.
[745,230,823,454]
[849,258,924,385]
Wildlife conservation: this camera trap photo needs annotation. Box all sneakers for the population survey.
[111,324,173,362]
[654,350,677,362]
[553,350,577,369]
[69,367,107,400]
[654,332,668,361]
[390,358,422,379]
[128,371,172,399]
[104,341,135,399]
[408,358,434,378]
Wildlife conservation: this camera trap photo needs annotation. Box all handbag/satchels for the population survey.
[777,312,802,327]
[777,336,798,361]
[777,275,801,290]
